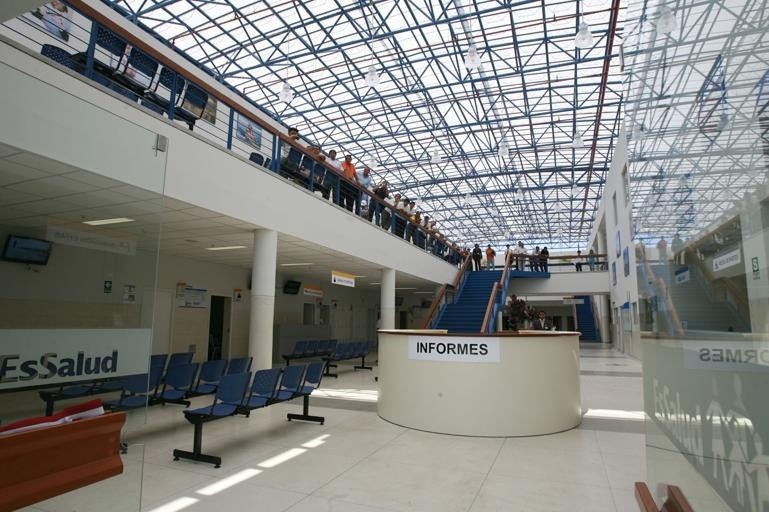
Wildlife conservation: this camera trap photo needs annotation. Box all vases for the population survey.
[508,319,521,331]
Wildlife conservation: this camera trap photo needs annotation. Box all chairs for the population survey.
[41,339,374,470]
[71,26,209,132]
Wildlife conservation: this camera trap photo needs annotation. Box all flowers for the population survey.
[498,295,537,324]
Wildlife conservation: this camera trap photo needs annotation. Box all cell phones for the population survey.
[420,219,422,221]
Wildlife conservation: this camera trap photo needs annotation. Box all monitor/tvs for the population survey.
[283,280,301,295]
[1,234,54,266]
[395,297,404,306]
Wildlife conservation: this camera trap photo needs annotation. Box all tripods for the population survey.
[422,301,432,308]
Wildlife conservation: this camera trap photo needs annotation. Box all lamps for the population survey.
[279,0,730,238]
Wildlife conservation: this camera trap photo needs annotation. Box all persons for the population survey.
[505,241,527,271]
[586,250,594,272]
[671,234,683,265]
[657,236,667,265]
[530,247,541,272]
[575,251,582,271]
[280,127,496,271]
[635,238,646,261]
[540,247,549,272]
[532,311,550,330]
